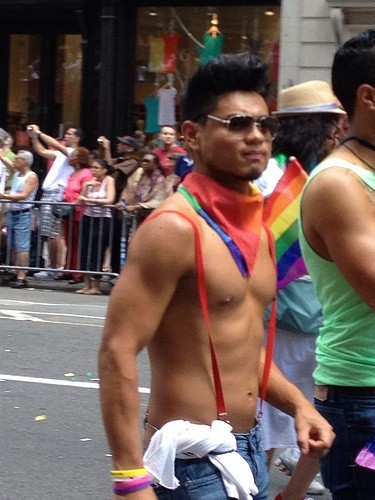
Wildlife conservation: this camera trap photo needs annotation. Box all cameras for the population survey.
[24,126,32,131]
[96,139,103,144]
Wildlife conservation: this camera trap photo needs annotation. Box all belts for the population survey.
[9,208,30,215]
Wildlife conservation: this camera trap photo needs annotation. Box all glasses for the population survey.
[206,114,280,134]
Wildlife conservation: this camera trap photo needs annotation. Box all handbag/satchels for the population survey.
[51,200,72,219]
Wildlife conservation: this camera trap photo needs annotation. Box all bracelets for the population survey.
[36,131,42,137]
[110,469,151,497]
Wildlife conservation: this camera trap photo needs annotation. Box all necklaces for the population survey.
[340,135,375,170]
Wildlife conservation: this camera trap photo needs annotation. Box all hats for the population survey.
[116,136,141,150]
[270,80,347,115]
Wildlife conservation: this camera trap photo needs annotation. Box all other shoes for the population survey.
[9,278,27,288]
[275,447,325,495]
[83,278,102,294]
[33,268,55,278]
[75,274,92,293]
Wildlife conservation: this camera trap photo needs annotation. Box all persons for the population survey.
[298,27,375,500]
[152,124,195,193]
[98,136,141,288]
[0,128,17,232]
[252,80,347,496]
[54,148,93,285]
[98,59,336,500]
[116,152,176,259]
[75,158,116,295]
[27,124,82,280]
[0,150,38,289]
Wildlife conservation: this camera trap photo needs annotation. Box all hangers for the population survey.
[149,79,173,96]
[207,25,222,34]
